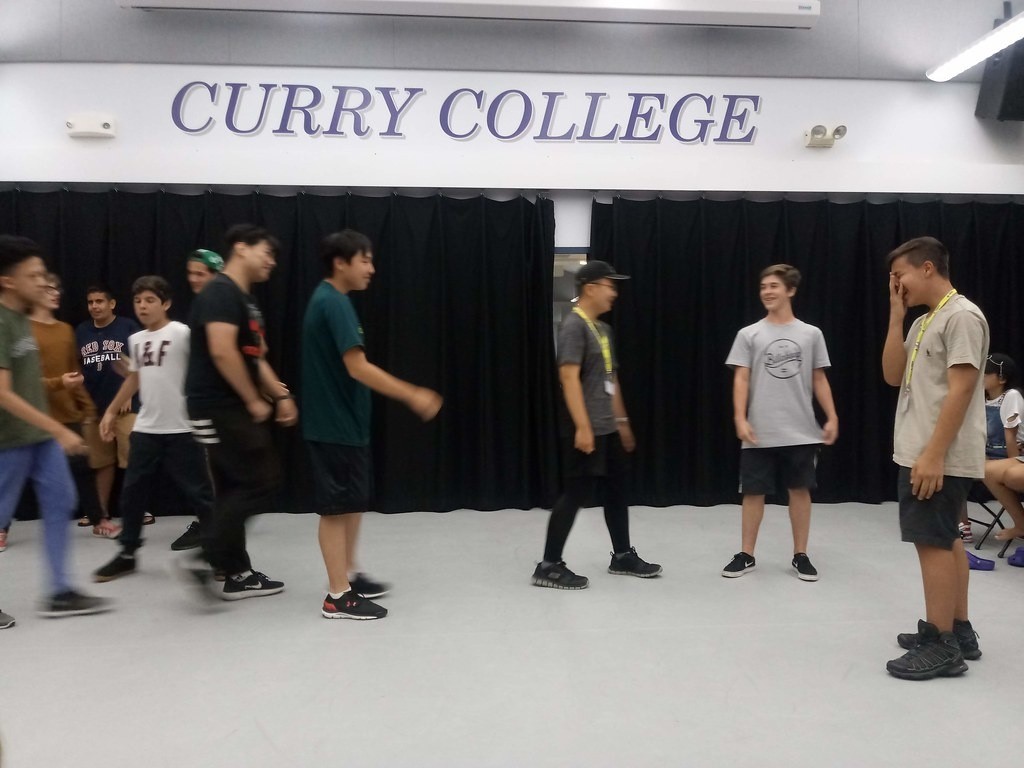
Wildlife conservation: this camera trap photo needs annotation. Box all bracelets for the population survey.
[273,395,292,403]
[616,417,628,421]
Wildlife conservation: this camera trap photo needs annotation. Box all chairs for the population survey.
[967,482,1022,558]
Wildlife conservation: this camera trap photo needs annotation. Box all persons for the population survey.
[75,285,151,523]
[0,275,124,552]
[958,356,1024,541]
[532,260,663,590]
[90,277,226,582]
[883,236,989,678]
[308,238,441,620]
[183,229,295,597]
[982,455,1024,540]
[171,253,266,551]
[0,245,115,628]
[722,264,838,580]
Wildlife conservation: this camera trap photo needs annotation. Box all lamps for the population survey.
[68,111,116,142]
[804,123,848,149]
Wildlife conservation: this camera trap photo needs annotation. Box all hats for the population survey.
[187,248,224,272]
[984,353,1015,374]
[575,260,630,279]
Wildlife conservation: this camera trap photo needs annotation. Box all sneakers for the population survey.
[321,590,387,620]
[792,553,818,581]
[722,552,756,577]
[171,521,203,550]
[897,619,982,660]
[608,545,662,578]
[886,620,968,681]
[531,562,589,589]
[349,573,389,598]
[223,569,284,601]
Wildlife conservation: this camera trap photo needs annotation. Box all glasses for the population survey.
[45,286,66,295]
[590,283,618,292]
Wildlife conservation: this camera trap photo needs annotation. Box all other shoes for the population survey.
[143,512,155,525]
[97,557,135,581]
[91,521,123,536]
[78,516,90,526]
[958,521,973,542]
[35,591,117,618]
[0,609,15,629]
[0,530,7,552]
